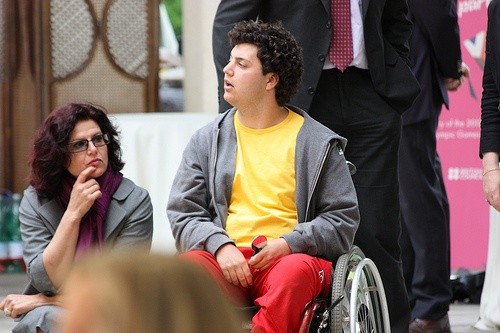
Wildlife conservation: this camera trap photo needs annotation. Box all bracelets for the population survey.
[482,168,500,176]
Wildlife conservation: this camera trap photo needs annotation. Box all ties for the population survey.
[328,0,354,72]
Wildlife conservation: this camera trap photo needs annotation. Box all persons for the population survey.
[167,22,359,333]
[478,0,500,212]
[0,103,152,333]
[213,0,420,333]
[369,1,469,333]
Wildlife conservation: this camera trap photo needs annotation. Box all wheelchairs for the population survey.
[296,160,392,333]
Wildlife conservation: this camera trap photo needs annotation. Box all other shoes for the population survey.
[408,312,452,333]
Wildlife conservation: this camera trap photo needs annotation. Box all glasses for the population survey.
[66,134,110,153]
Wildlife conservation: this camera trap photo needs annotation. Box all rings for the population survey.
[4,308,10,314]
[486,199,491,206]
